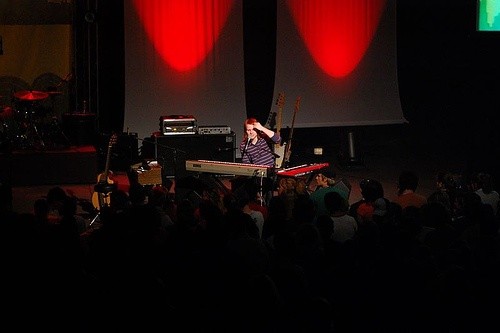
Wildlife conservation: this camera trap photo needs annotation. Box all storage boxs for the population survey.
[154,116,236,178]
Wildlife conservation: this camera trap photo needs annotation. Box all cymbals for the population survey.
[14,88,49,103]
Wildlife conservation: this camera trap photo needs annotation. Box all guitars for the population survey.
[263,91,302,166]
[90,134,119,211]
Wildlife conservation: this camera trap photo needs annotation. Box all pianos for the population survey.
[185,156,271,181]
[275,160,331,180]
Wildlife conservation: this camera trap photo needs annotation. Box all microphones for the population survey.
[245,138,251,151]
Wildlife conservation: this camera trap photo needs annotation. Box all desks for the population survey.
[0,145,99,187]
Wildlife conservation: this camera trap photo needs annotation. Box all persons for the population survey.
[324,192,358,238]
[349,177,402,223]
[393,172,427,209]
[239,117,282,193]
[0,173,500,333]
[310,166,350,208]
